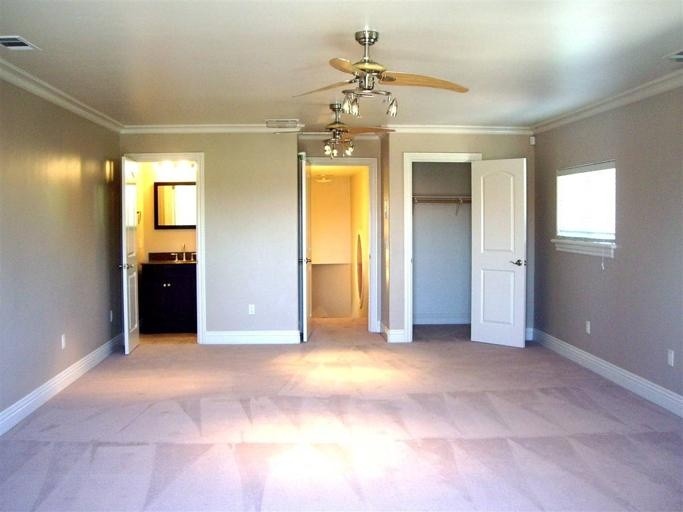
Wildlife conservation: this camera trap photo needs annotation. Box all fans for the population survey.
[296,103,396,132]
[293,30,468,98]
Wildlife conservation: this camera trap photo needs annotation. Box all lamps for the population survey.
[342,72,397,116]
[324,131,353,159]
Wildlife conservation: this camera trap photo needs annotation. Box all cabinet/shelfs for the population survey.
[138,264,197,333]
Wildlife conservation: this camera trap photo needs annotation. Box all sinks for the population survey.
[174,260,196,263]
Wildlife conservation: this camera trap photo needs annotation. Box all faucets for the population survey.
[182,243,186,261]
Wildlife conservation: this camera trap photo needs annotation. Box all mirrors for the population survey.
[154,182,197,229]
